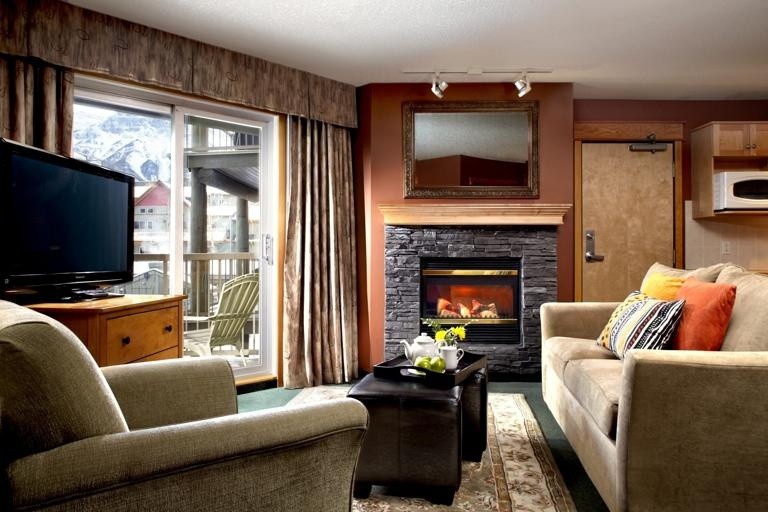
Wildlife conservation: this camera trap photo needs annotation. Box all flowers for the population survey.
[420,318,468,346]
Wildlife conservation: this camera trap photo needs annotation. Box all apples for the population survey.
[414,356,445,374]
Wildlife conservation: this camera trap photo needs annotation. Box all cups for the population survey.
[440,346,464,370]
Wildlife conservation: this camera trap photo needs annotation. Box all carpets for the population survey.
[284,386,576,512]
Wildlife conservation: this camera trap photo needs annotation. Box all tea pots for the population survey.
[401,333,447,365]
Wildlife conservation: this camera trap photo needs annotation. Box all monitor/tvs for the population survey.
[0,137,135,301]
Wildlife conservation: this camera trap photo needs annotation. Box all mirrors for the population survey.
[401,99,541,199]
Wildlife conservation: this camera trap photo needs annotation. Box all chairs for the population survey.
[184,274,258,368]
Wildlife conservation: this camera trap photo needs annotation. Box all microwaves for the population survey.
[713,171,768,210]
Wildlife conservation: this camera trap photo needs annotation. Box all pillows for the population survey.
[596,290,686,361]
[673,275,737,350]
[715,265,767,351]
[639,257,732,300]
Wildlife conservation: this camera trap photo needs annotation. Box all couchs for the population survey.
[538,263,768,511]
[0,291,371,511]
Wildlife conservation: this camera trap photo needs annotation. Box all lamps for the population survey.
[514,71,532,98]
[431,72,448,98]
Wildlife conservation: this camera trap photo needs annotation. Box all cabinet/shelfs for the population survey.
[691,122,767,219]
[28,293,188,368]
